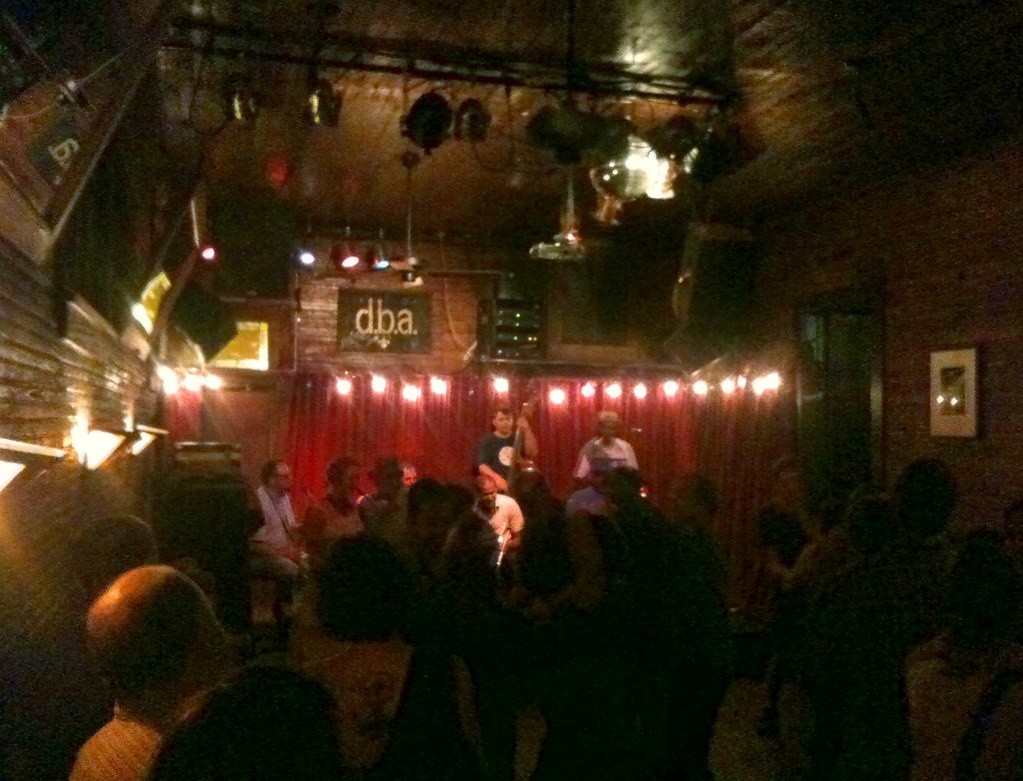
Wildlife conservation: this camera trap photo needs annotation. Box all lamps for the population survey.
[228,92,256,120]
[310,83,339,125]
[372,243,389,269]
[401,152,423,290]
[336,242,358,268]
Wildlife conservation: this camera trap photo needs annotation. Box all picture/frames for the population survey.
[928,344,982,441]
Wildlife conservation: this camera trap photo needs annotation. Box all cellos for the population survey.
[506,393,543,500]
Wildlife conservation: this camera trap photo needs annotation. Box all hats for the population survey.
[368,457,404,478]
[596,411,621,425]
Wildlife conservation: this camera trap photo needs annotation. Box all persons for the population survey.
[757,459,1023,781]
[30,457,736,781]
[565,410,647,511]
[483,406,539,493]
